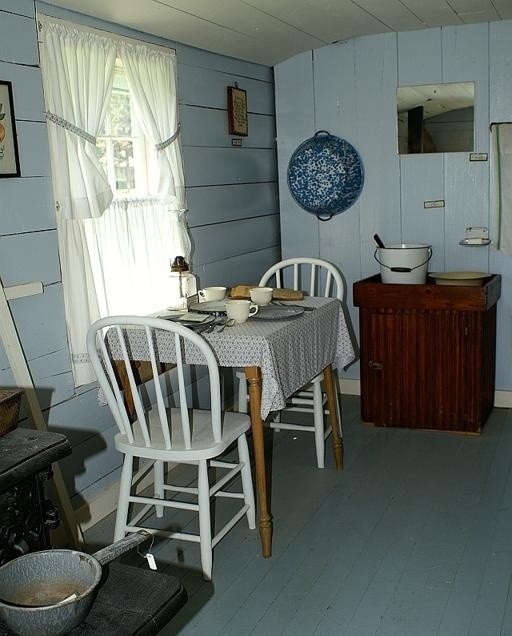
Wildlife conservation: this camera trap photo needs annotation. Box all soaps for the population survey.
[466,237,482,244]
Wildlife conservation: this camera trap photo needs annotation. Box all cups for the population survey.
[198,286,273,324]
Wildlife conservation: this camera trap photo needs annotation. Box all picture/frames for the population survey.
[0,80,20,178]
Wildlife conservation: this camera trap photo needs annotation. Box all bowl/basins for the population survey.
[427,271,493,286]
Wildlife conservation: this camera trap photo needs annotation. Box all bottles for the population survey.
[164,255,198,311]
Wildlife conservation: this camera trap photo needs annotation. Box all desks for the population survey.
[353,272,501,434]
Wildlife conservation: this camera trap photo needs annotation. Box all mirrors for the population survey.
[396,81,474,154]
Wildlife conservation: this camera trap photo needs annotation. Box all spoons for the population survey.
[215,318,235,333]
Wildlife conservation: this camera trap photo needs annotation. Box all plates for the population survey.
[189,299,230,315]
[163,314,215,327]
[253,305,306,319]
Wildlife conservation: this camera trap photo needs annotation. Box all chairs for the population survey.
[86,315,251,581]
[235,257,344,468]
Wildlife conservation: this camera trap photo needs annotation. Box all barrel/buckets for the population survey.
[373,244,432,286]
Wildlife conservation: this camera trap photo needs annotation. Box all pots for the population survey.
[287,131,365,222]
[1,530,150,635]
